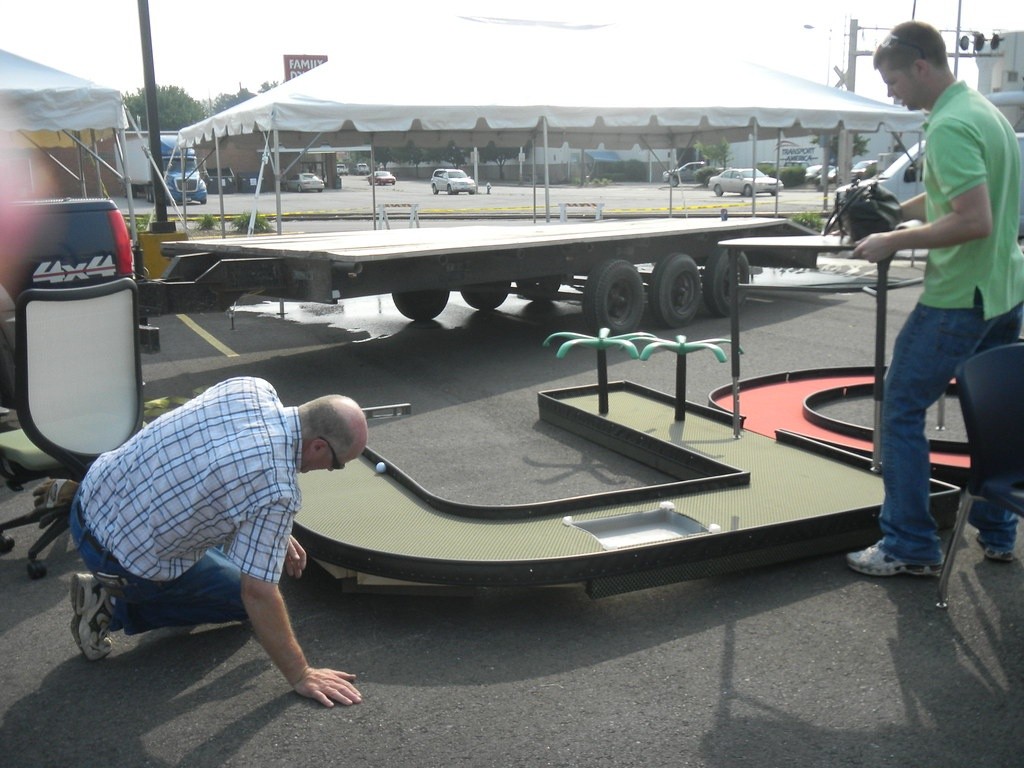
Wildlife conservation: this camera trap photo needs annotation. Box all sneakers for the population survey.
[70,572,125,661]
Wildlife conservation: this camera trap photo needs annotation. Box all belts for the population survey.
[75,500,120,564]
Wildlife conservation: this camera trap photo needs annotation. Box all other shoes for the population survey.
[845,543,945,576]
[982,546,1015,562]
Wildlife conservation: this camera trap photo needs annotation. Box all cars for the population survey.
[285,173,324,193]
[783,159,879,186]
[708,168,783,197]
[368,171,397,186]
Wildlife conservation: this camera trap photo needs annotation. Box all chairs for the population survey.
[0,279,145,580]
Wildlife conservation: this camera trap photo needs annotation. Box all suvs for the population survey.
[431,167,476,196]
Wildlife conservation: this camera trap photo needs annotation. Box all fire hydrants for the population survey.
[486,182,492,195]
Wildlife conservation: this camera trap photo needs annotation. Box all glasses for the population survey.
[317,436,346,470]
[875,31,926,61]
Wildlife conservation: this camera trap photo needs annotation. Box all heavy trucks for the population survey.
[114,134,207,206]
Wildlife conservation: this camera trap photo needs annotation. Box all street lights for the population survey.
[803,23,832,88]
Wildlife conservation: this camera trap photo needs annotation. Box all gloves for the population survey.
[32,478,80,529]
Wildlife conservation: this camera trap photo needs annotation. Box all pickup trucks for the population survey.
[662,161,733,187]
[336,163,349,177]
[354,163,370,175]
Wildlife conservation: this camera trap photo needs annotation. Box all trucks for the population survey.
[836,90,1024,244]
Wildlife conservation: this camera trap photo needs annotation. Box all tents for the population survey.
[177,21,951,316]
[2,47,140,247]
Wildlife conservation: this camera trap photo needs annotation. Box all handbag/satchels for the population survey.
[821,178,903,243]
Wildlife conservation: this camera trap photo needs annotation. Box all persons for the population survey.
[842,22,1024,576]
[70,377,369,709]
